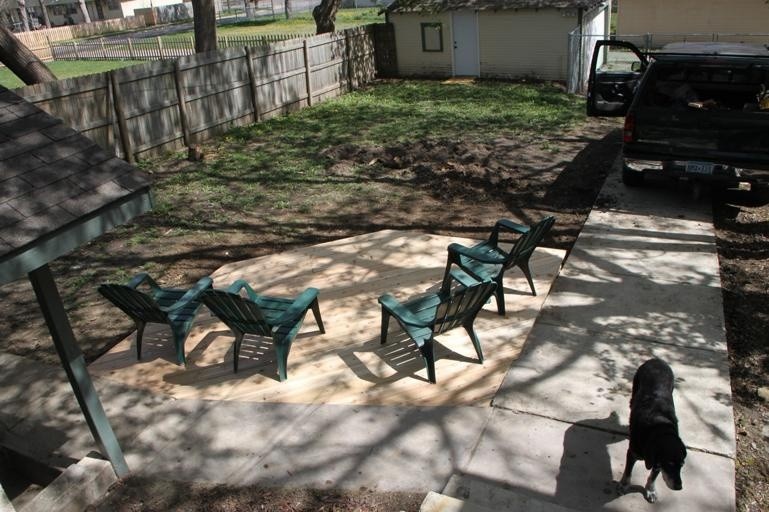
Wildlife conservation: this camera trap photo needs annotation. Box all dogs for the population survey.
[616,358,687,504]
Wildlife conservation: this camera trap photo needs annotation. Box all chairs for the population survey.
[98,273,215,365]
[442,215,554,314]
[377,269,498,385]
[199,279,327,382]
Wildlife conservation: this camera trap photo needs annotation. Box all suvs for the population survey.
[587,40,768,207]
